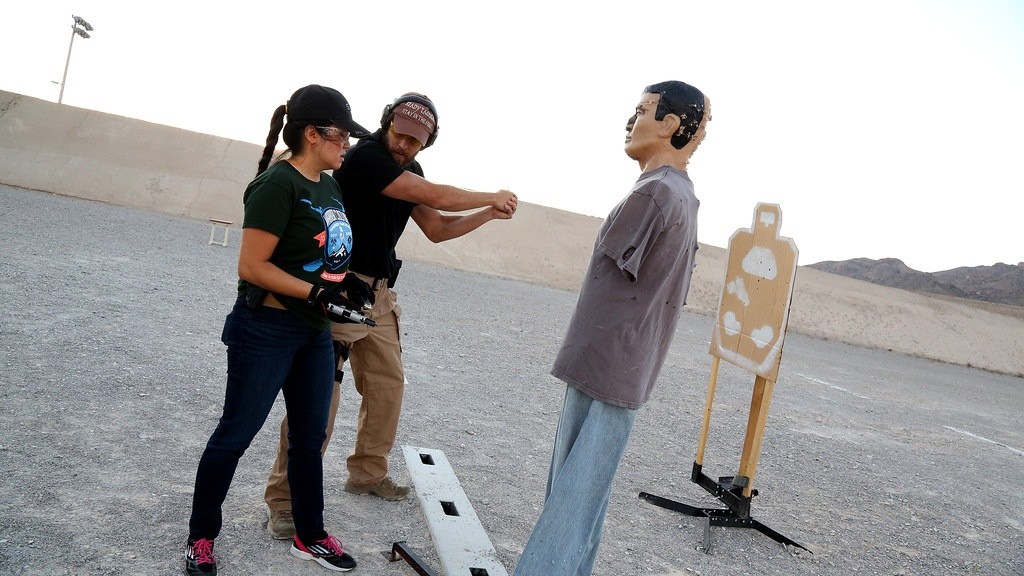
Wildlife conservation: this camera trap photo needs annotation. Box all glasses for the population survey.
[390,121,422,147]
[315,125,350,143]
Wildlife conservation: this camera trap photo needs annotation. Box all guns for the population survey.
[328,299,378,328]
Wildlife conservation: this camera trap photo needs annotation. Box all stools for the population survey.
[208,218,233,247]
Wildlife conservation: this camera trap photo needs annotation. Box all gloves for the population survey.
[314,289,364,324]
[341,272,375,307]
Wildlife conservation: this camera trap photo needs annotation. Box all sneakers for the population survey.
[185,538,217,576]
[267,507,296,539]
[345,476,411,501]
[290,534,357,571]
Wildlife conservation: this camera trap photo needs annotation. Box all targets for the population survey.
[707,201,799,382]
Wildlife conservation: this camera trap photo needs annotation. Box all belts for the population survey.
[347,270,388,292]
[245,290,289,310]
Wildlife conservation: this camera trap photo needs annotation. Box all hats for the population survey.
[287,85,371,138]
[391,92,436,147]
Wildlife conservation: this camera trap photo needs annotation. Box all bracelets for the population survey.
[309,283,321,303]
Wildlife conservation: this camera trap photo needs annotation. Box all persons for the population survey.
[511,80,711,576]
[186,86,375,576]
[266,93,517,538]
